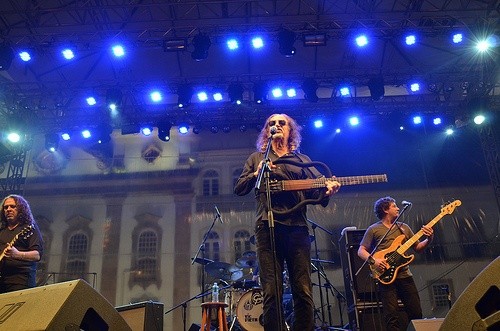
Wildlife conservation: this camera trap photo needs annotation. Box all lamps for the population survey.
[0,24,491,152]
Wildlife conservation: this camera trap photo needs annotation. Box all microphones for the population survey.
[401,201,411,205]
[270,126,276,134]
[215,206,224,223]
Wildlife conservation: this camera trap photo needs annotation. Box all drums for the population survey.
[217,288,246,321]
[235,287,266,331]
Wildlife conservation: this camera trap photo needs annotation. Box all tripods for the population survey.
[307,218,349,331]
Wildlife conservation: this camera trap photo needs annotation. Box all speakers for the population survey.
[339,229,403,310]
[0,279,133,331]
[349,303,411,331]
[407,318,445,331]
[114,301,164,331]
[438,256,500,331]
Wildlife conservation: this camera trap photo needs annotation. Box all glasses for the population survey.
[269,121,285,126]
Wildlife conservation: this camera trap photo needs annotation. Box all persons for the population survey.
[234,113,340,331]
[0,194,43,294]
[357,197,435,331]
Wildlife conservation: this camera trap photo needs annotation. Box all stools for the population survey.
[200,301,229,331]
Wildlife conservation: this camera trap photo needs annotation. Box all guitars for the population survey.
[370,198,462,285]
[258,158,391,218]
[0,223,35,263]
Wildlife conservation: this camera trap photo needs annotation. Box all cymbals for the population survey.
[309,232,316,244]
[236,254,258,268]
[249,234,256,246]
[310,258,335,264]
[204,261,243,282]
[190,256,214,266]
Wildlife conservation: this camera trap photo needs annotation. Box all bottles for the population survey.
[212,283,219,302]
